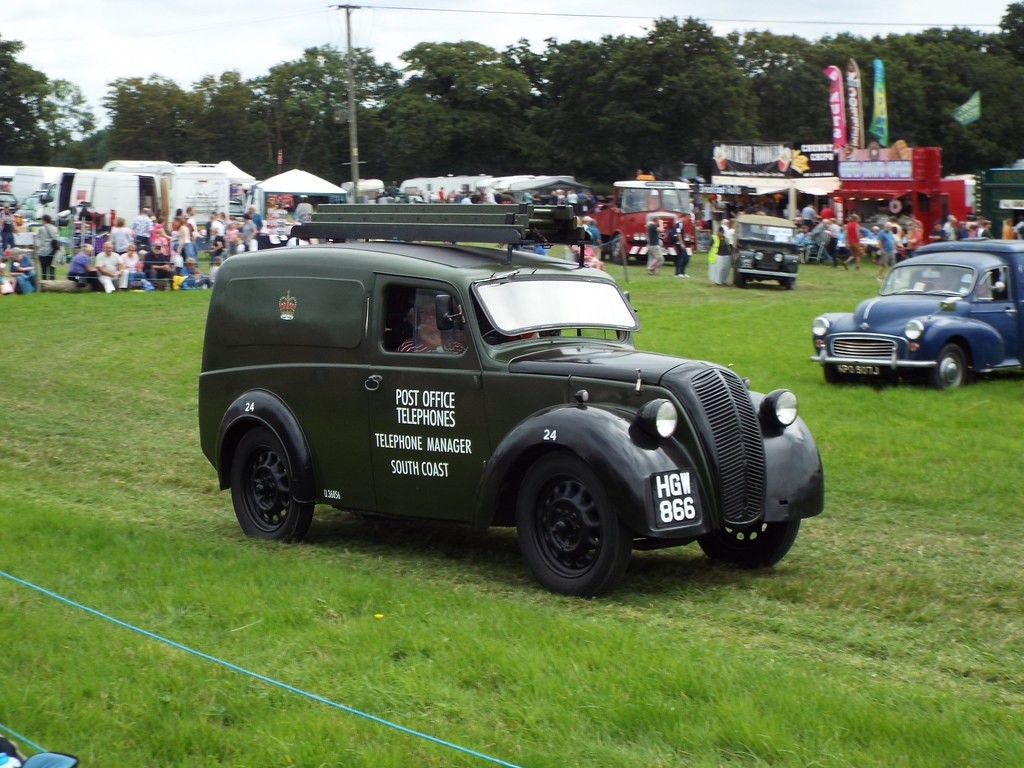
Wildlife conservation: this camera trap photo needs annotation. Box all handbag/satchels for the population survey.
[51,240,60,250]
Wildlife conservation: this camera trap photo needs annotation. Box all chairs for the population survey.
[382,302,414,351]
[960,274,973,293]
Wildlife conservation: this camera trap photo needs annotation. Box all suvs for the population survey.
[197,204,828,599]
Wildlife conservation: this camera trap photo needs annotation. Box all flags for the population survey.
[824,66,845,148]
[951,91,981,125]
[868,60,888,147]
[845,58,864,150]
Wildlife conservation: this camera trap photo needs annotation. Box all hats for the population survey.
[852,214,861,221]
[184,213,190,218]
[151,243,162,246]
[81,244,94,250]
[718,226,724,233]
[10,249,25,256]
[934,221,941,224]
[983,220,991,224]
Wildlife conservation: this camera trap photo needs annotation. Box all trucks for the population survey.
[808,235,1024,391]
[585,174,699,266]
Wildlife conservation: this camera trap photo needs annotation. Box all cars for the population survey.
[729,210,809,289]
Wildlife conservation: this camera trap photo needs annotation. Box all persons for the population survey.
[439,187,501,204]
[396,293,465,354]
[522,188,613,270]
[707,227,731,284]
[68,198,313,293]
[928,214,1024,242]
[645,213,691,278]
[0,203,61,294]
[795,204,923,280]
[691,192,785,246]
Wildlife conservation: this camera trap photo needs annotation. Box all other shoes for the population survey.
[674,274,685,278]
[685,274,690,278]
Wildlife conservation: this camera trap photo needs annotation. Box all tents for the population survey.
[255,169,347,221]
